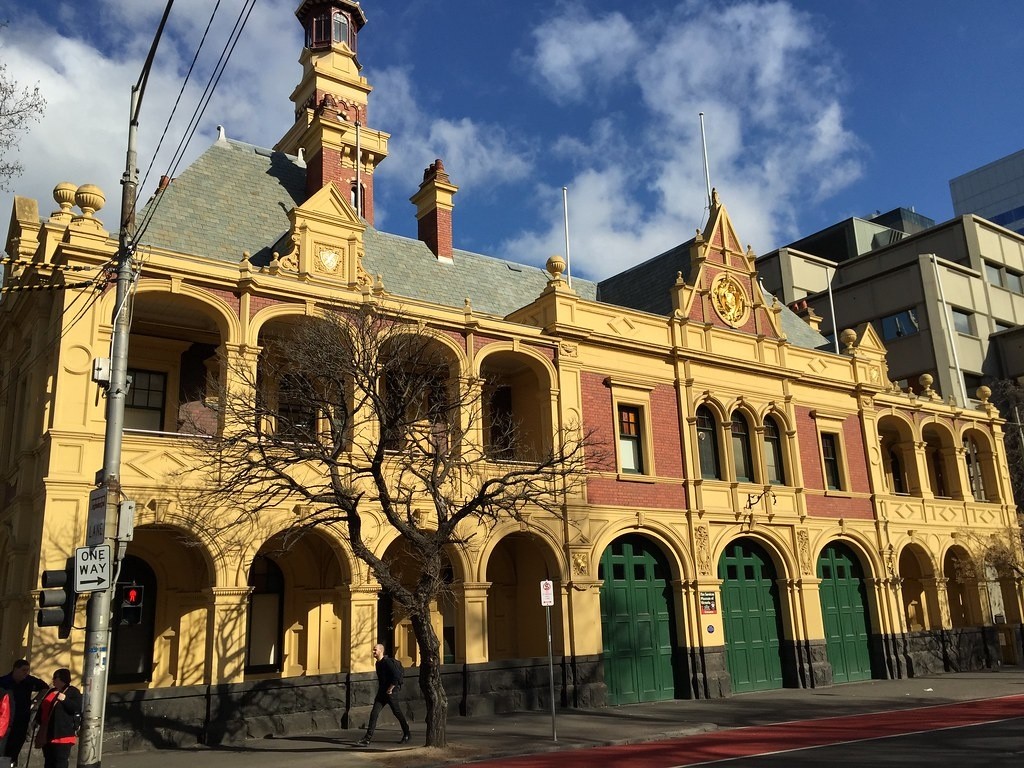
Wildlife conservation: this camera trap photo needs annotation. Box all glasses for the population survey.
[54,675,60,679]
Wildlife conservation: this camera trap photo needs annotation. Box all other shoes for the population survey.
[355,738,370,746]
[397,735,410,744]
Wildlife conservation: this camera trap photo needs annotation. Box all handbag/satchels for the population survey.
[35,726,48,749]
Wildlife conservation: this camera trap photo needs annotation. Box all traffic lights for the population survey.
[121,586,144,626]
[38,557,81,639]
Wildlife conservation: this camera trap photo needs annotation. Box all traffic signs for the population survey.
[74,546,110,592]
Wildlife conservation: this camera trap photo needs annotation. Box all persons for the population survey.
[31,668,82,768]
[0,659,50,768]
[355,644,412,747]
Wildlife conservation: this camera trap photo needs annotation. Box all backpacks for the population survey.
[380,657,404,691]
